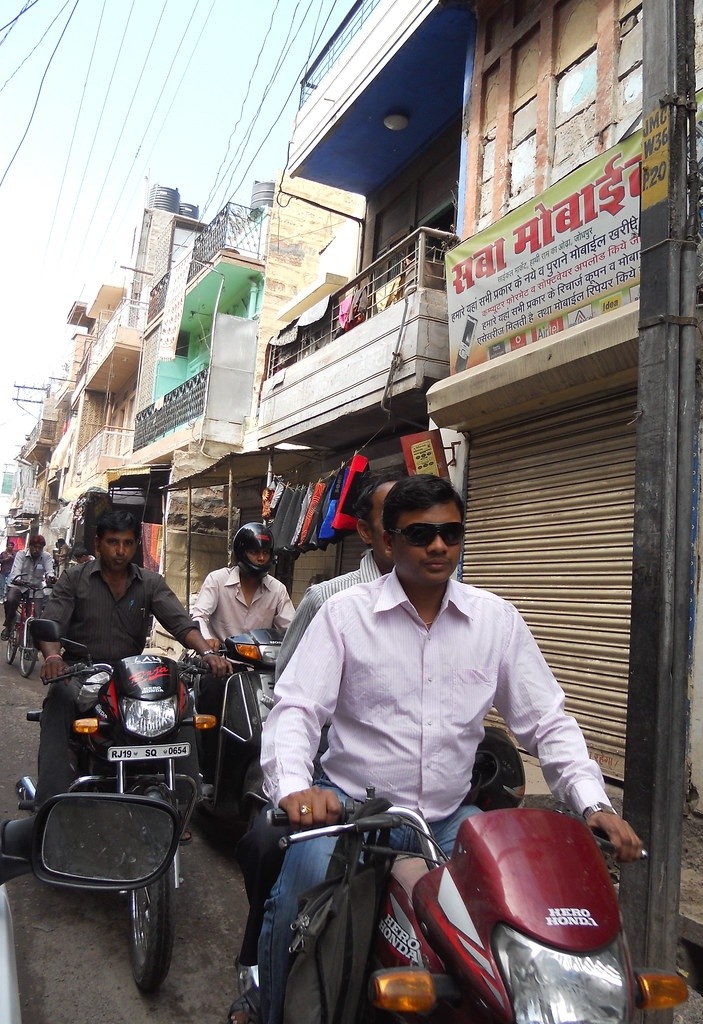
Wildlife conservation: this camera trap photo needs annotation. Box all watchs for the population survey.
[584,802,617,819]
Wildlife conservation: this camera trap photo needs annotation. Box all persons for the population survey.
[34,509,228,845]
[229,473,643,1024]
[192,522,295,798]
[1,535,75,641]
[75,547,95,563]
[0,542,16,603]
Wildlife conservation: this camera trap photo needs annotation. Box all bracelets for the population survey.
[201,648,213,657]
[46,654,62,661]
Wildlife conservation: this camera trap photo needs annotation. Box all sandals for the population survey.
[228,995,259,1024]
[179,825,193,846]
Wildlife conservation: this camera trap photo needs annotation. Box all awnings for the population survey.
[160,450,317,489]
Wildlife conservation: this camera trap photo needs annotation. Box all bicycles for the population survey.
[5,573,53,678]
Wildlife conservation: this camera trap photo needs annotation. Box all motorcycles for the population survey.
[231,782,690,1024]
[14,618,255,996]
[195,626,288,835]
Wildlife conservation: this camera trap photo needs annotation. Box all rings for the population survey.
[300,805,312,815]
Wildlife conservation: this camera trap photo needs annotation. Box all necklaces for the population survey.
[426,622,432,625]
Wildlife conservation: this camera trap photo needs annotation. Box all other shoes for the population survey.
[1,626,13,641]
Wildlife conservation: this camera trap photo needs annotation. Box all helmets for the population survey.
[233,522,278,579]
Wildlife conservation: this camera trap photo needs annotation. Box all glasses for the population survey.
[389,521,465,546]
